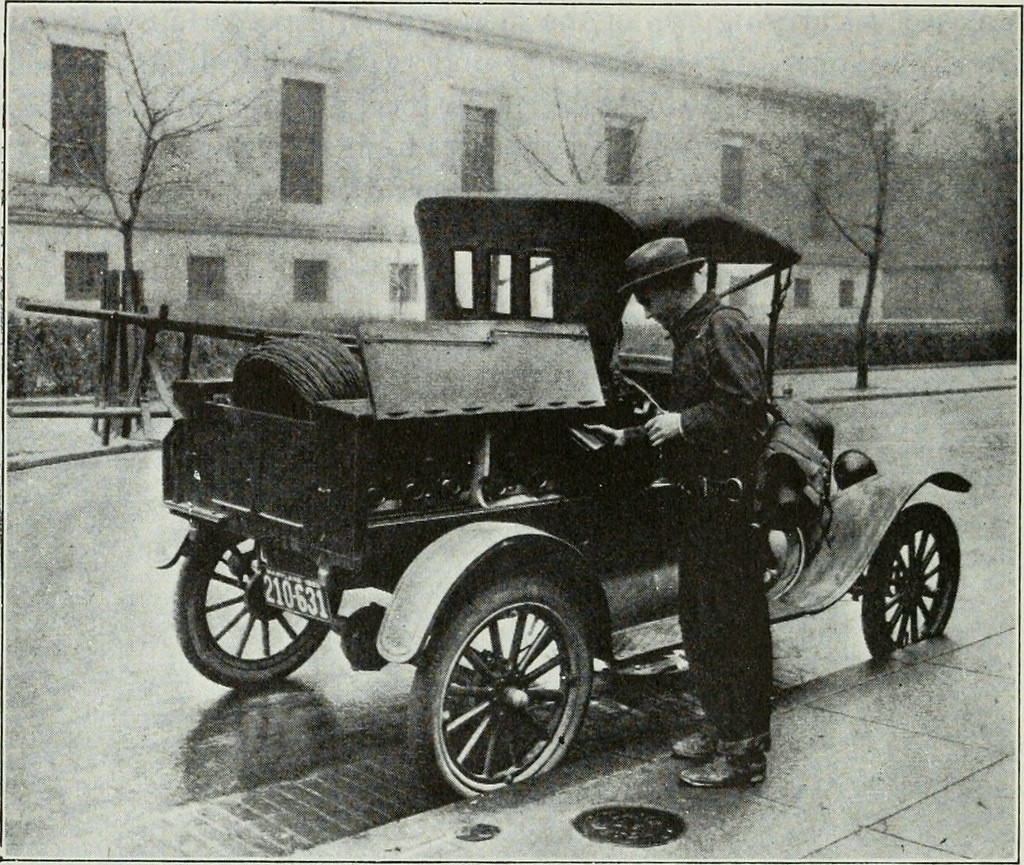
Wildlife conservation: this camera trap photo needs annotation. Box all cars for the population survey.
[149,172,977,807]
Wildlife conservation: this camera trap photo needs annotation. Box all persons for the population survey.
[584,238,773,785]
